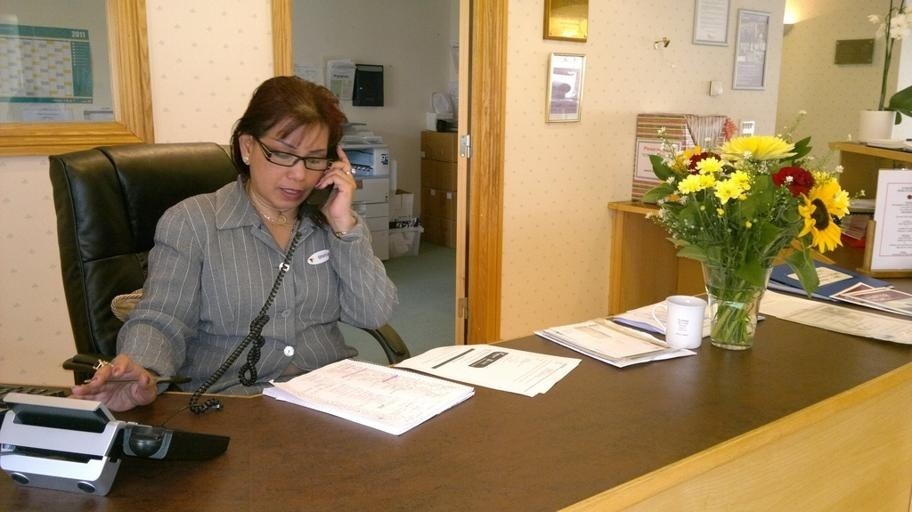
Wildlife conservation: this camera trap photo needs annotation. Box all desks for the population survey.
[0,253,911,512]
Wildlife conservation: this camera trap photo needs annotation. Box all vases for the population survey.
[860,109,897,148]
[702,262,771,349]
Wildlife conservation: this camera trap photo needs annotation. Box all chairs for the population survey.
[52,142,421,409]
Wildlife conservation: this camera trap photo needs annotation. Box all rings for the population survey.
[345,168,357,176]
[91,357,113,368]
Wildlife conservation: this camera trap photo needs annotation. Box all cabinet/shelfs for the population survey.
[827,134,910,277]
[339,142,391,264]
[417,132,458,251]
[599,196,705,316]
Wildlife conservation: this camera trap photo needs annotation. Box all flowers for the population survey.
[646,111,852,349]
[861,0,912,123]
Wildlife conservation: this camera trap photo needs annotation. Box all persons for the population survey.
[66,74,398,415]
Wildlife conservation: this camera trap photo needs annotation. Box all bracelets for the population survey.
[335,219,358,236]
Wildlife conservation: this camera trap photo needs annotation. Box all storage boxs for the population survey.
[391,188,414,223]
[630,110,728,204]
[386,225,425,258]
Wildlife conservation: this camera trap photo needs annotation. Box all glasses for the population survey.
[252,134,336,171]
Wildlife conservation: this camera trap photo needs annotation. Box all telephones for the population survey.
[297,185,333,216]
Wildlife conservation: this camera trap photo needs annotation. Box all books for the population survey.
[264,358,477,437]
[633,113,726,205]
[337,122,383,145]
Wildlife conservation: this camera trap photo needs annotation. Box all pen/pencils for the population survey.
[84,377,192,384]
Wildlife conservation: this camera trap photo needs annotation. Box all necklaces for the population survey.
[260,207,290,227]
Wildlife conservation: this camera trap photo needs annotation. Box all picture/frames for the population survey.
[691,0,733,53]
[540,0,590,44]
[733,6,773,91]
[544,50,588,125]
[0,0,159,164]
